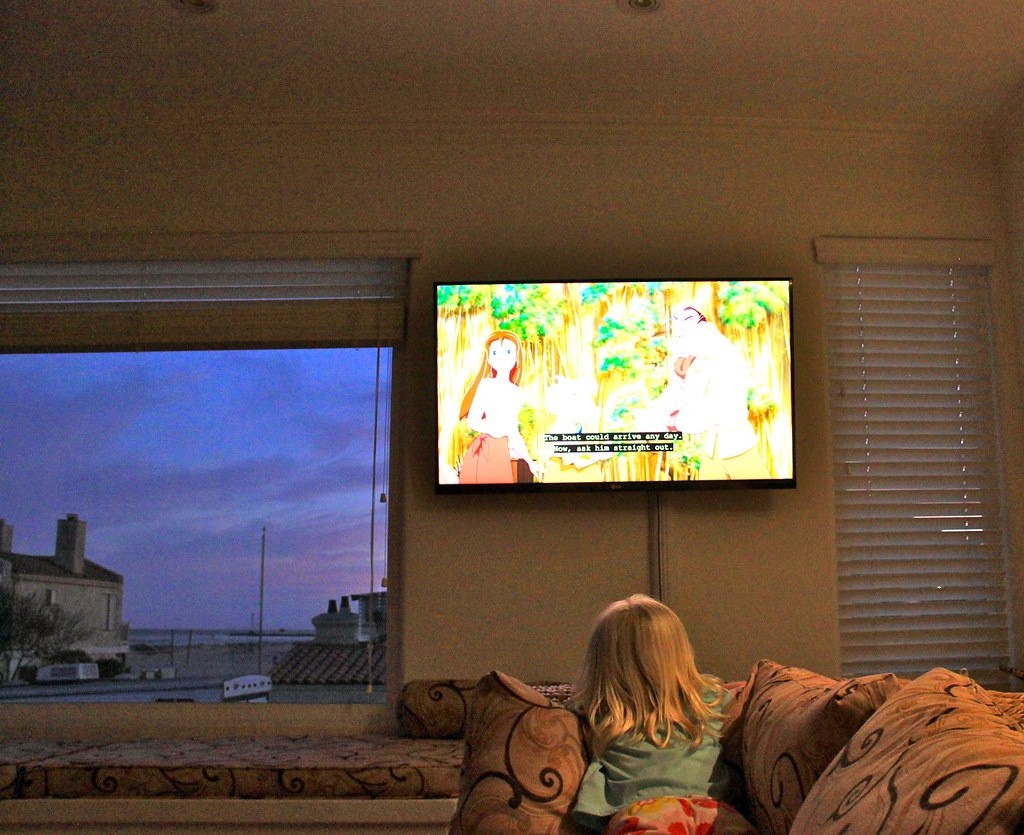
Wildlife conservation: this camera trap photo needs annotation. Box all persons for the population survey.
[568,591,756,835]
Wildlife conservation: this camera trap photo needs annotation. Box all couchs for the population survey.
[1,704,1024,834]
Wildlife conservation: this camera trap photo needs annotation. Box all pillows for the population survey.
[742,660,902,835]
[788,668,1024,835]
[396,679,566,739]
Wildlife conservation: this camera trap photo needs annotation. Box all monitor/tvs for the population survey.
[432,276,797,495]
